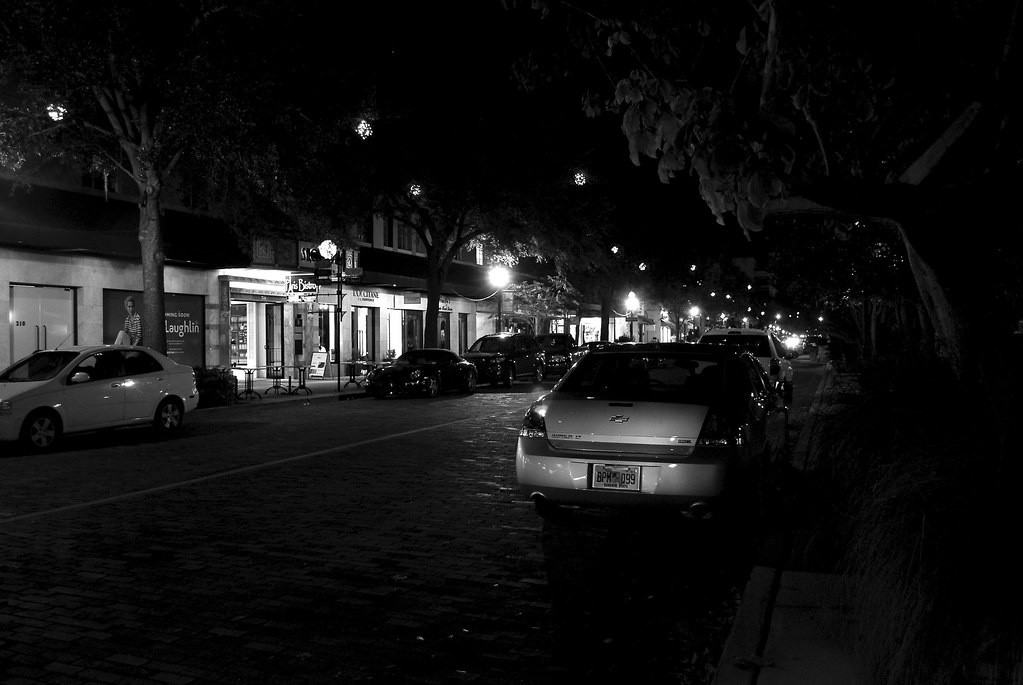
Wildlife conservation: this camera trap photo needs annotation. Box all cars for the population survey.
[534,335,584,378]
[579,341,612,362]
[516,343,781,528]
[0,345,200,452]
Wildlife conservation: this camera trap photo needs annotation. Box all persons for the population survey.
[114,296,142,347]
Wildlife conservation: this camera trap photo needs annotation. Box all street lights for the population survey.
[622,290,642,340]
[689,306,700,342]
[486,263,513,330]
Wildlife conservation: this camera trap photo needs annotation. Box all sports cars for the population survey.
[364,347,480,398]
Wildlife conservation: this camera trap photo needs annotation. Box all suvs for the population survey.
[692,326,799,405]
[461,332,547,388]
